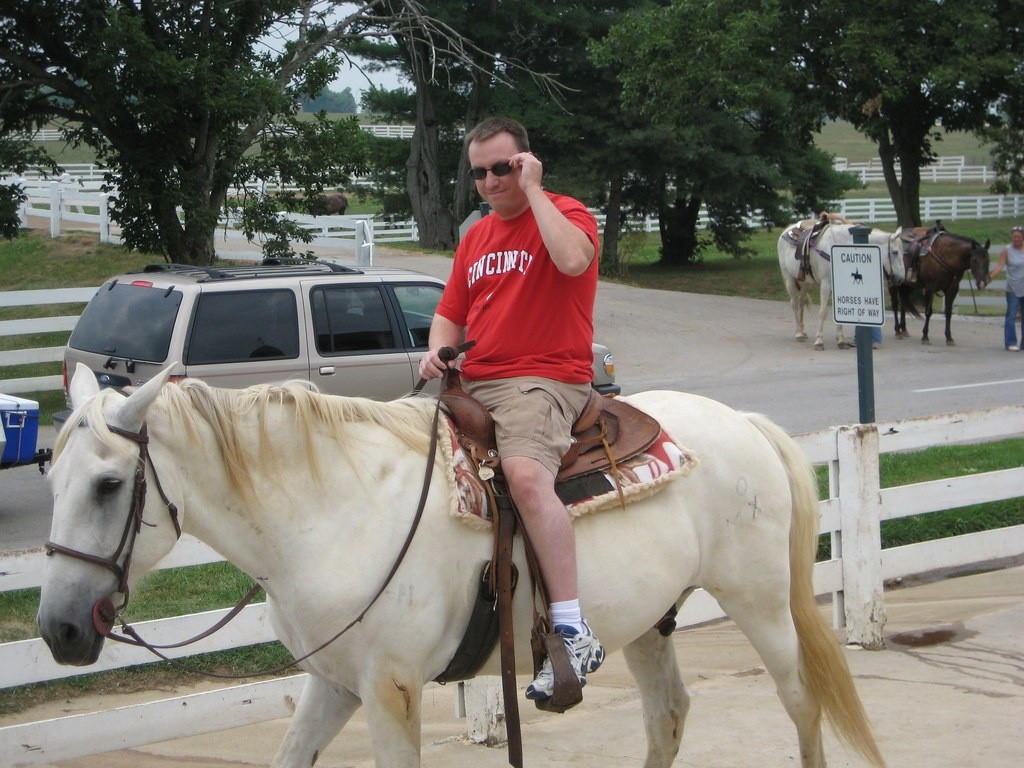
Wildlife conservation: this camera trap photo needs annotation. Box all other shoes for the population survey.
[848,337,856,347]
[1006,345,1019,351]
[872,340,881,350]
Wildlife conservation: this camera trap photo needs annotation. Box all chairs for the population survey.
[361,297,396,350]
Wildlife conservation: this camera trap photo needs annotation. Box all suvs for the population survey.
[52,255,621,436]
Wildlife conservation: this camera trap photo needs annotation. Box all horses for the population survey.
[33,361,888,768]
[776,219,991,351]
[310,195,348,215]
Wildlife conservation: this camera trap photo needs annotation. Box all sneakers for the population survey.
[525,618,606,701]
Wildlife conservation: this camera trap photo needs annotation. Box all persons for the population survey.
[990,227,1024,351]
[419,116,607,700]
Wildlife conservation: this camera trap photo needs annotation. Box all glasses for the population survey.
[1012,226,1024,231]
[467,162,512,180]
